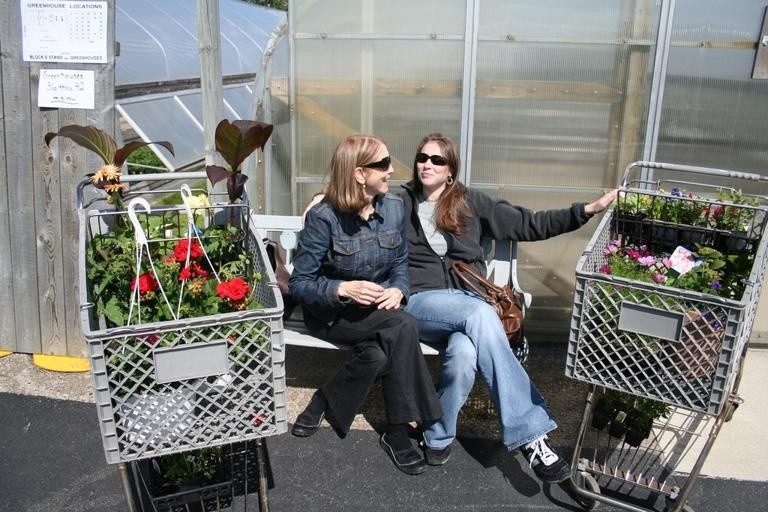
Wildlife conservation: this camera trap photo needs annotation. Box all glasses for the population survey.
[358,157,392,170]
[415,153,448,167]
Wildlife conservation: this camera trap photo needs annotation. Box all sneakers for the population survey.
[291,407,327,437]
[520,438,572,483]
[380,431,425,474]
[424,441,452,465]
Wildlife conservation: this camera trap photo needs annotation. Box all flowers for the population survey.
[596,185,766,303]
[86,181,257,389]
[583,187,768,350]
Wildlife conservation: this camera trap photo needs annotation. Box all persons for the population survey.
[287,134,444,475]
[301,133,625,484]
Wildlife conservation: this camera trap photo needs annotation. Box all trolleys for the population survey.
[563,160,768,512]
[76,168,287,512]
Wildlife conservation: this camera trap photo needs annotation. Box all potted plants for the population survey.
[148,444,227,495]
[592,388,673,448]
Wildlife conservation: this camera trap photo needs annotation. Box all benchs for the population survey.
[249,212,538,419]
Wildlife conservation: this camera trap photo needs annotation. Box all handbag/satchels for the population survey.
[452,260,519,340]
[263,238,301,320]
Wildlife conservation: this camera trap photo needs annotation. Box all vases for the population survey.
[603,308,728,381]
[111,390,199,448]
